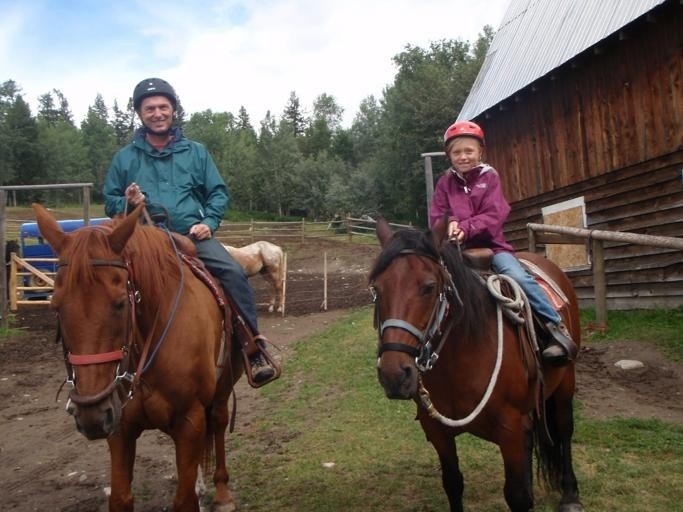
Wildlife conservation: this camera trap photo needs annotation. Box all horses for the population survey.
[5,238,21,304]
[31,201,247,512]
[368,210,584,512]
[219,239,285,313]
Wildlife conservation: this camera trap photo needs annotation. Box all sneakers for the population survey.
[542,322,581,360]
[242,336,276,386]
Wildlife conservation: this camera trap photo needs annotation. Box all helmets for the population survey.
[133,77,176,112]
[444,121,485,147]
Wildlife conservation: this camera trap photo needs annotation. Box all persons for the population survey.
[428,121,576,359]
[100,77,275,383]
